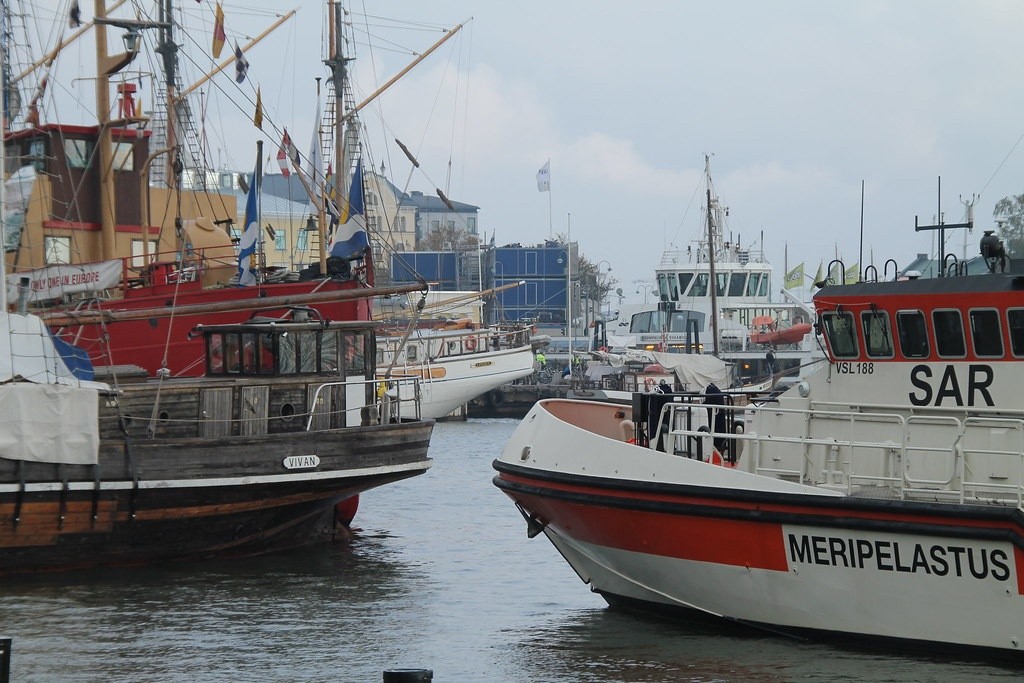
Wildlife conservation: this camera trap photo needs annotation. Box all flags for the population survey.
[213,5,370,287]
[489,232,495,248]
[536,161,550,192]
[784,262,858,292]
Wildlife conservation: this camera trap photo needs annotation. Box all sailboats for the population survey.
[0,0,437,577]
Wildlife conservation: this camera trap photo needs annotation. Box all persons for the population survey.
[659,379,672,394]
[614,411,635,443]
[766,349,775,375]
[491,332,581,368]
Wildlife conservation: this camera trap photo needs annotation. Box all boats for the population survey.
[341,268,541,430]
[492,176,1023,656]
[452,154,833,421]
